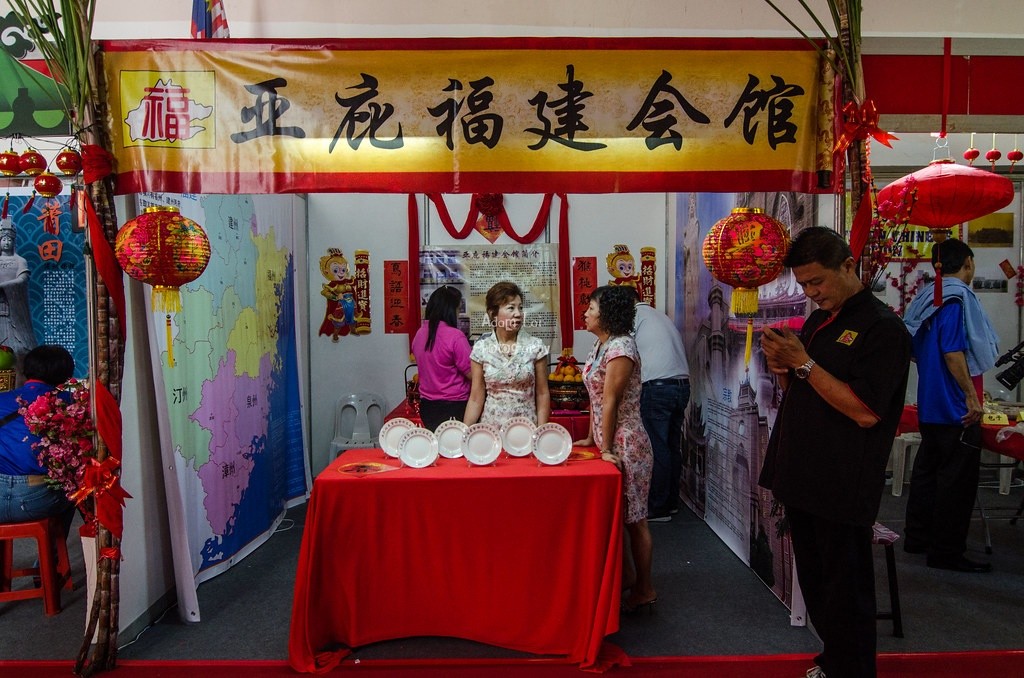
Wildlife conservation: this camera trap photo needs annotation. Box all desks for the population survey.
[311,447,623,654]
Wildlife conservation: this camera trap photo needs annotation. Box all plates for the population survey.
[397,428,439,469]
[499,417,537,457]
[379,418,416,457]
[434,420,470,458]
[461,423,503,465]
[532,423,572,465]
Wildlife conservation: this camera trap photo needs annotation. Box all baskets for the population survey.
[546,362,590,410]
[404,364,420,412]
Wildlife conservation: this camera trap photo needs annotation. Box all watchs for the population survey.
[794,359,816,379]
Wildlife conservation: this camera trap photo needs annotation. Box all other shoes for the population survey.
[926,553,993,574]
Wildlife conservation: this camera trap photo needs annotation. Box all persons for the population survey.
[411,286,475,430]
[903,237,999,573]
[761,226,911,677]
[463,280,550,431]
[0,344,78,589]
[0,214,38,372]
[629,286,692,521]
[572,284,660,605]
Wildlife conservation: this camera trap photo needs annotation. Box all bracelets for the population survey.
[600,450,612,454]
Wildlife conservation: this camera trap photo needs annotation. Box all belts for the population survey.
[641,377,690,387]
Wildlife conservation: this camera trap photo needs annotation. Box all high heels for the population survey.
[619,597,657,615]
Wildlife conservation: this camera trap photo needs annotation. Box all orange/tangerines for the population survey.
[413,374,418,382]
[548,366,582,381]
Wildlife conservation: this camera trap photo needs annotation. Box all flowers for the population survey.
[16,380,94,522]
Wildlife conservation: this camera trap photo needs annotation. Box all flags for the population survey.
[191,0,231,39]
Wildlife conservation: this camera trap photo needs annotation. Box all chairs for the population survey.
[327,389,389,462]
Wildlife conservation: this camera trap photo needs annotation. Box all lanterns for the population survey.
[0,150,23,219]
[56,148,83,211]
[19,150,47,214]
[985,149,1002,173]
[116,207,210,369]
[962,148,980,167]
[1007,149,1023,174]
[33,171,64,235]
[874,156,1014,308]
[702,207,791,369]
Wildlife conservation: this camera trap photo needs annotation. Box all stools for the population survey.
[0,516,74,614]
[871,523,904,638]
[891,432,921,496]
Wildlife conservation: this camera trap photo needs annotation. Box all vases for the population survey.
[82,527,100,643]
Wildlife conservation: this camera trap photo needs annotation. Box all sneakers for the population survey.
[645,506,678,522]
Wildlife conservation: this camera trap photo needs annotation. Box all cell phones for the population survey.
[763,328,784,340]
